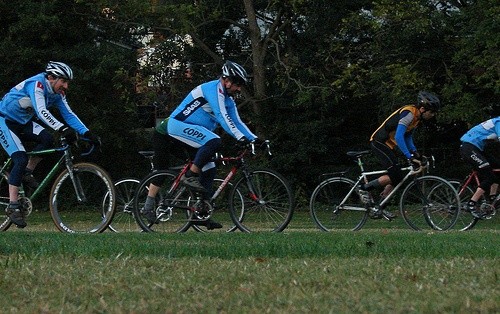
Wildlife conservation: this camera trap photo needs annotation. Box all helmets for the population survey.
[222,59,249,86]
[418,90,440,112]
[46,60,73,81]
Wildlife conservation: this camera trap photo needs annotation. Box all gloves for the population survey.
[83,131,102,149]
[238,137,251,149]
[253,138,264,144]
[59,125,77,144]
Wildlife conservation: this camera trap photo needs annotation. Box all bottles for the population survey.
[380,184,394,199]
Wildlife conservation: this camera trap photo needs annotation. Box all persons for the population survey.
[354,90,441,218]
[141,61,264,234]
[459,116,500,220]
[0,60,99,229]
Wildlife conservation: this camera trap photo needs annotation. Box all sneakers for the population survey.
[465,201,483,218]
[180,174,209,193]
[22,173,39,188]
[358,181,373,206]
[197,219,223,229]
[383,209,396,218]
[140,207,160,225]
[4,204,27,228]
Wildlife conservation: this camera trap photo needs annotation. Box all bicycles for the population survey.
[0,127,118,235]
[132,138,296,235]
[99,150,246,234]
[309,150,462,232]
[423,144,500,231]
